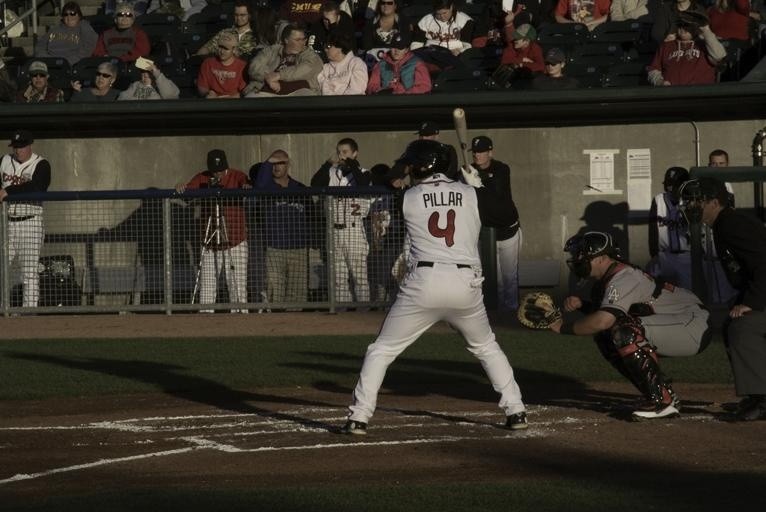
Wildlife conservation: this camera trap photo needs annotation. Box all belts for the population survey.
[417,261,470,269]
[333,222,355,229]
[7,215,34,222]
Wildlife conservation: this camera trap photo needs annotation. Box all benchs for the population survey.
[6,253,460,317]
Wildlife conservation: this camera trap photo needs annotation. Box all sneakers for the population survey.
[506,412,528,430]
[632,393,682,422]
[336,421,369,437]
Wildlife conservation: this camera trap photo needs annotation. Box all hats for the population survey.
[28,61,49,77]
[511,23,537,42]
[542,47,565,63]
[207,149,228,172]
[7,129,34,148]
[414,120,439,137]
[466,135,493,152]
[217,32,236,50]
[389,32,410,46]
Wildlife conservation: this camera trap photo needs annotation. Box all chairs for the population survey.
[0,0,766,104]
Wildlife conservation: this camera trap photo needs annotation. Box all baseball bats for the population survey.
[454,108,470,172]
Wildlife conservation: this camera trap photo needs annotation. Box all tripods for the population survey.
[190,188,242,314]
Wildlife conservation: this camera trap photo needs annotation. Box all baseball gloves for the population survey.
[519,292,560,329]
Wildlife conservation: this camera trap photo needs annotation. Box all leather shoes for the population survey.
[716,396,766,421]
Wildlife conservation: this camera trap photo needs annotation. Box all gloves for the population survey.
[460,164,486,189]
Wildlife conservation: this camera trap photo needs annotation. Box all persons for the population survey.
[702,150,738,305]
[458,136,520,311]
[1,0,766,106]
[678,176,766,423]
[0,129,51,317]
[243,150,315,312]
[367,120,457,311]
[176,149,250,314]
[649,167,713,293]
[310,138,370,312]
[544,229,714,421]
[338,152,529,434]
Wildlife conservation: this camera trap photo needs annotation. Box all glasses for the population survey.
[116,11,133,17]
[322,44,334,49]
[94,70,111,79]
[391,43,406,50]
[546,62,558,66]
[379,1,394,6]
[61,11,78,17]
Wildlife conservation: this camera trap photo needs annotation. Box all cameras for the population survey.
[209,176,221,186]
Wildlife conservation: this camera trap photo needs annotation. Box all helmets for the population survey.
[564,228,615,276]
[664,177,728,230]
[395,140,449,180]
